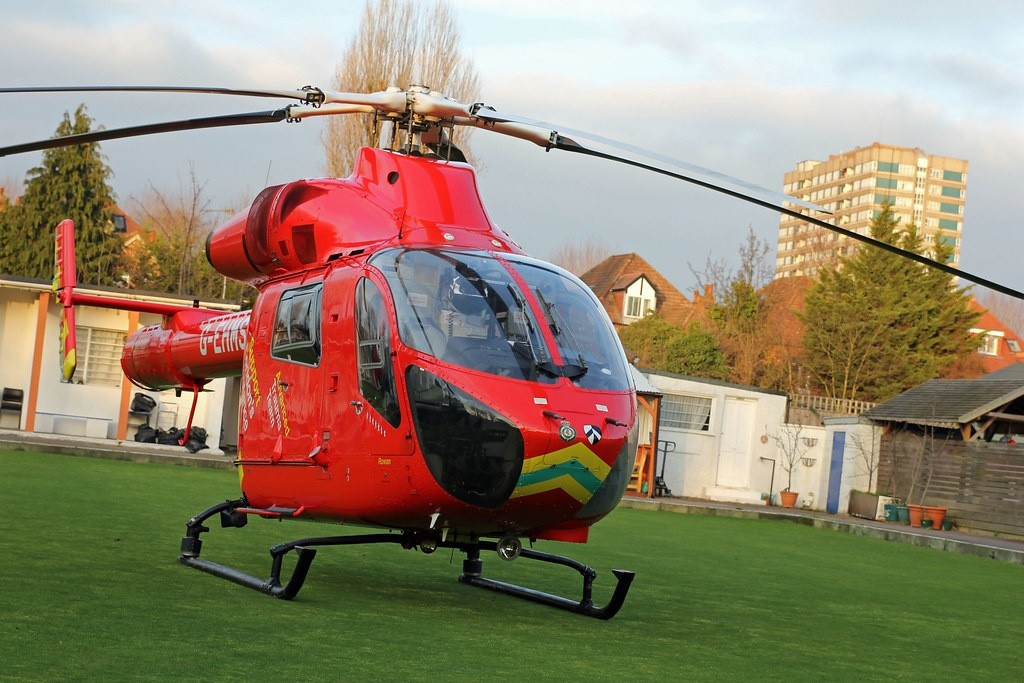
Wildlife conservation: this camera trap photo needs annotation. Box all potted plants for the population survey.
[843,404,955,530]
[767,420,811,508]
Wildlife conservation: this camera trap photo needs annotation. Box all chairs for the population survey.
[627,451,647,493]
[0,387,23,429]
[125,410,152,439]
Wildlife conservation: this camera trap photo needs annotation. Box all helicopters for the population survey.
[0,82,1024,620]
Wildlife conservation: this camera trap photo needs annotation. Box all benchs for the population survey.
[35,411,114,439]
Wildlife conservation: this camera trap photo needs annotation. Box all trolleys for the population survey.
[655,440,677,497]
